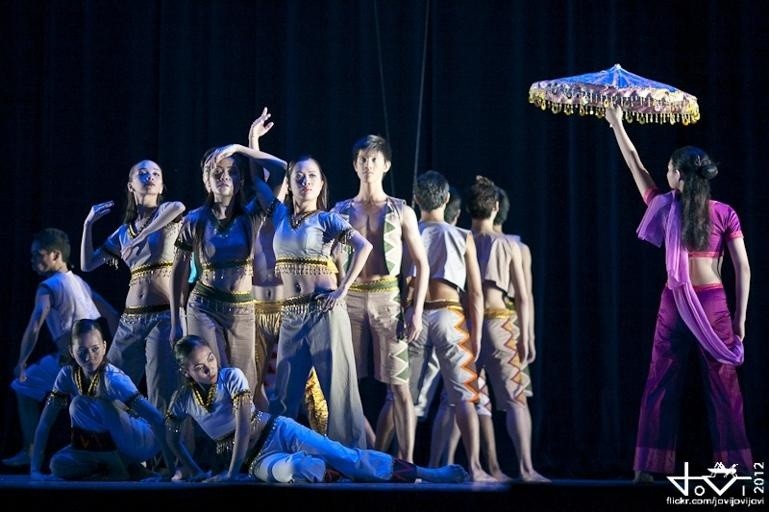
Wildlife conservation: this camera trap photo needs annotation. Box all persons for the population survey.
[607,104,754,482]
